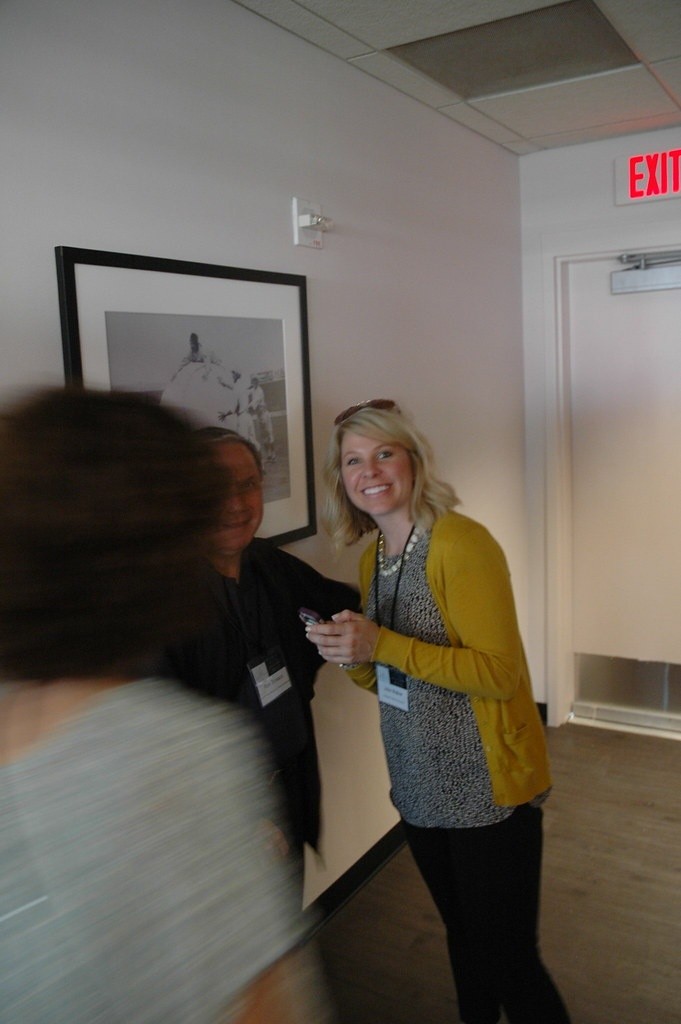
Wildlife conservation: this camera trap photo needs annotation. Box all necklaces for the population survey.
[378,524,424,577]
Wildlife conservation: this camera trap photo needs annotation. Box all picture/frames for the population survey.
[54,244,317,548]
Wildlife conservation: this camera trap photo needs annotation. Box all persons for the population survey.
[0,387,320,1024]
[302,398,571,1024]
[134,330,272,463]
[150,426,364,907]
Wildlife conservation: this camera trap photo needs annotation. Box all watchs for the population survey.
[338,662,359,672]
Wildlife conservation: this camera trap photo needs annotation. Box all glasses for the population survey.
[334,398,399,425]
